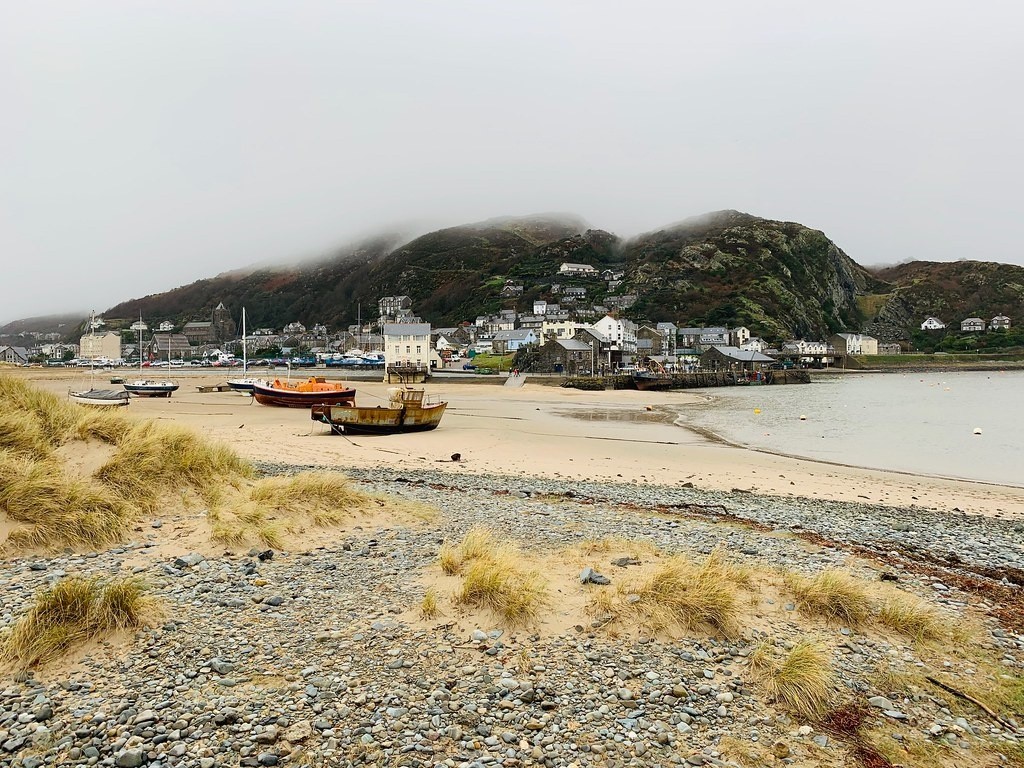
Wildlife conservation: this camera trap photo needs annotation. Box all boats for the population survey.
[68,310,129,407]
[253,376,356,407]
[312,387,448,429]
[632,375,672,391]
[226,307,266,393]
[124,309,179,396]
[110,377,125,385]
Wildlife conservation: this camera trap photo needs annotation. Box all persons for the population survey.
[508,367,521,378]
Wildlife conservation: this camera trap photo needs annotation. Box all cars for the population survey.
[768,364,784,370]
[463,361,478,370]
[65,352,316,369]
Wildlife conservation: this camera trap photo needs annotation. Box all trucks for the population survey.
[442,355,461,363]
